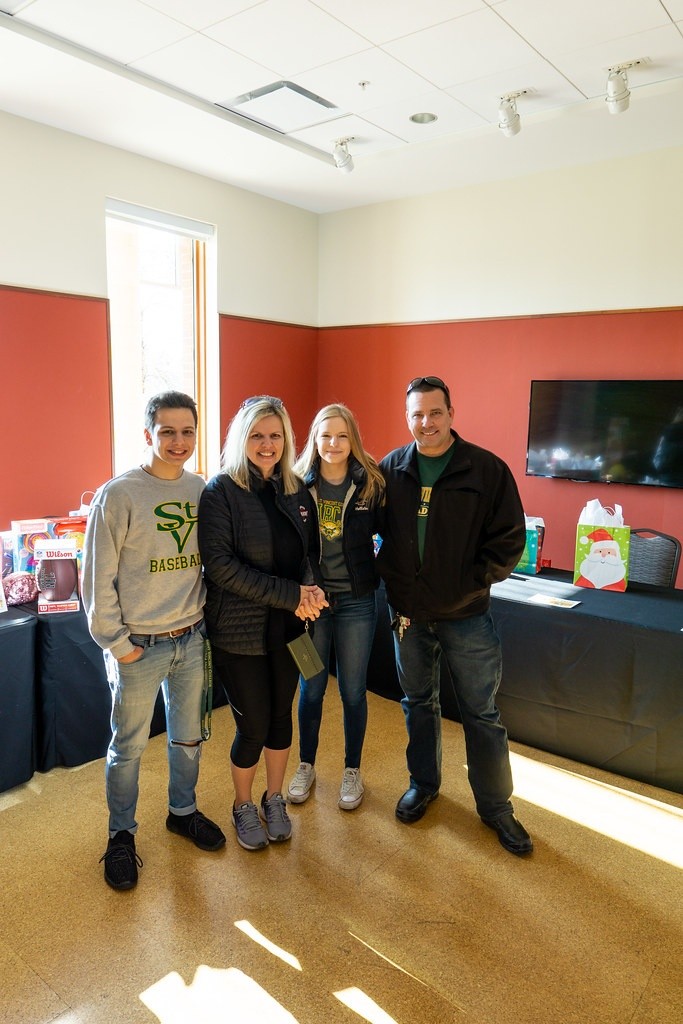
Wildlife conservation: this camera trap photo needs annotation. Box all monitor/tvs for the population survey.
[526,379,683,489]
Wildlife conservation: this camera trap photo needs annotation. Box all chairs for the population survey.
[627,528,681,588]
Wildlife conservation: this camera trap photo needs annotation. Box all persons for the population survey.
[292,402,384,810]
[373,374,534,858]
[197,394,329,853]
[79,390,226,892]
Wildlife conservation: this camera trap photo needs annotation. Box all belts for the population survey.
[130,618,203,639]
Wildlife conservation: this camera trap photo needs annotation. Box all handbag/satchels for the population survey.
[511,525,544,575]
[286,618,325,680]
[572,506,630,593]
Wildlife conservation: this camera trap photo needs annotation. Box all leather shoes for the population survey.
[396,788,440,822]
[480,812,531,854]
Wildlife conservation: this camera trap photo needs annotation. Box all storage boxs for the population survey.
[0,515,88,580]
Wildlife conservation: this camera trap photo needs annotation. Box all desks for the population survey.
[311,568,683,796]
[0,575,229,792]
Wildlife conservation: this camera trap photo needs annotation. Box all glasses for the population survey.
[406,376,449,395]
[243,396,282,408]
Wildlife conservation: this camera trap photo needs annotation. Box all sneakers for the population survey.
[99,831,143,889]
[338,767,364,809]
[259,790,292,842]
[166,808,226,850]
[232,799,269,850]
[287,762,316,803]
